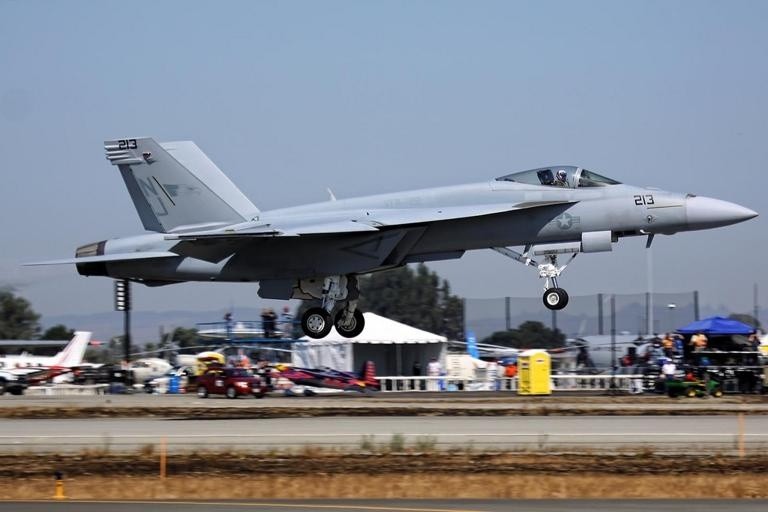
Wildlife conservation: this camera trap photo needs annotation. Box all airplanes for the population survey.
[1,331,107,395]
[22,137,759,340]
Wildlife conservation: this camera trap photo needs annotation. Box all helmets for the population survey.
[556,170,567,182]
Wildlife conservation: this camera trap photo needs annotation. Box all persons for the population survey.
[269,307,278,333]
[426,355,442,390]
[413,360,422,375]
[632,330,709,363]
[224,312,234,337]
[280,304,295,337]
[555,170,567,185]
[748,333,762,351]
[502,362,517,389]
[260,307,274,337]
